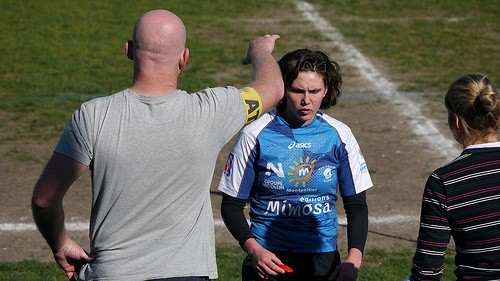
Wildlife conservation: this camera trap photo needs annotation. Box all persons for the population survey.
[406,71,500,281]
[31,10,284,281]
[217,48,375,281]
